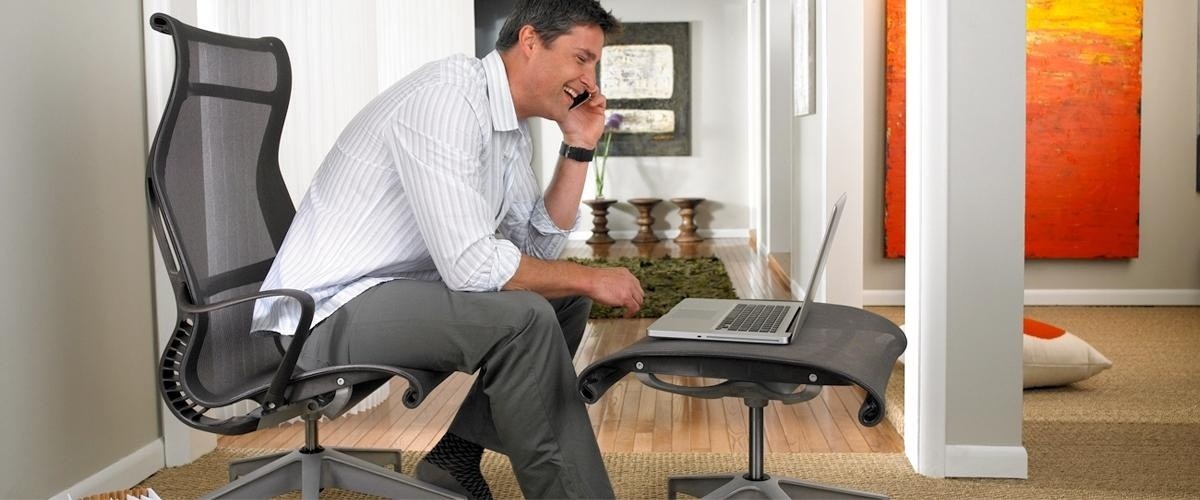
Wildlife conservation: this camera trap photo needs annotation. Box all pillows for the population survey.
[892,311,1111,390]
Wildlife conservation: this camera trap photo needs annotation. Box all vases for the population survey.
[584,200,616,244]
[627,198,663,245]
[670,198,706,242]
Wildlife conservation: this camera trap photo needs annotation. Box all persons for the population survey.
[246,0,647,500]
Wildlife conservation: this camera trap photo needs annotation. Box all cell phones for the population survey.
[569,89,591,111]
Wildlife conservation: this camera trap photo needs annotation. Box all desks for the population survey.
[568,298,905,499]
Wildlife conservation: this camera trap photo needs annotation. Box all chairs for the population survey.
[145,13,473,500]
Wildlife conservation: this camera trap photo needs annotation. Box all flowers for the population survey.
[593,113,625,200]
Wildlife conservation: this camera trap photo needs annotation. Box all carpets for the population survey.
[564,254,742,320]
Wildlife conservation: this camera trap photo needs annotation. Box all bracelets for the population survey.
[558,141,596,163]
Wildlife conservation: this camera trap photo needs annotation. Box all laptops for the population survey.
[646,193,847,345]
